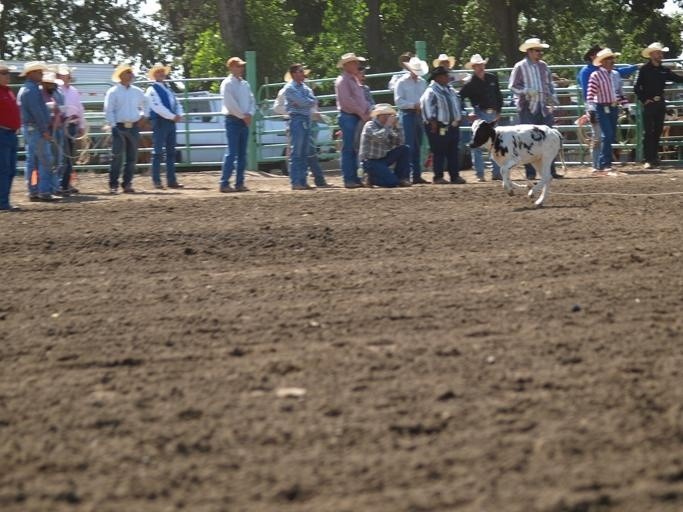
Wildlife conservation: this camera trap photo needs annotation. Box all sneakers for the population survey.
[157,185,166,189]
[643,162,652,168]
[167,184,183,188]
[492,176,503,180]
[220,186,235,192]
[345,174,372,188]
[110,188,119,195]
[319,184,333,188]
[400,176,465,186]
[7,188,78,211]
[124,189,133,193]
[477,177,485,181]
[292,185,311,189]
[234,187,249,192]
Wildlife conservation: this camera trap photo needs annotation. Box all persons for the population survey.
[1,60,87,212]
[509,38,564,179]
[102,62,186,192]
[334,51,504,188]
[272,63,330,190]
[578,40,682,172]
[219,56,256,192]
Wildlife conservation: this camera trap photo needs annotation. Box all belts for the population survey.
[117,123,134,128]
[649,96,660,102]
[602,103,616,107]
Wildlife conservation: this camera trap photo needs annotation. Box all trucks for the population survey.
[0,62,114,176]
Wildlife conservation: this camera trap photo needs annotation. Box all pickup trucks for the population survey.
[511,59,683,160]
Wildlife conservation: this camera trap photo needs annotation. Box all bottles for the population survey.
[355,162,364,179]
[574,112,589,127]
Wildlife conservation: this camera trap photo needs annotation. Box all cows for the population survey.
[468,118,568,209]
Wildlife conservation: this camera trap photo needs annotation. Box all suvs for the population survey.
[143,90,343,176]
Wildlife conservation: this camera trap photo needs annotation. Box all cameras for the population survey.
[392,116,399,121]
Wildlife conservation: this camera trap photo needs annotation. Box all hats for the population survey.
[641,42,669,58]
[148,62,171,80]
[583,43,621,66]
[337,53,365,67]
[0,60,76,86]
[519,38,550,52]
[111,63,133,83]
[403,54,488,76]
[370,104,397,117]
[226,57,247,68]
[284,70,310,81]
